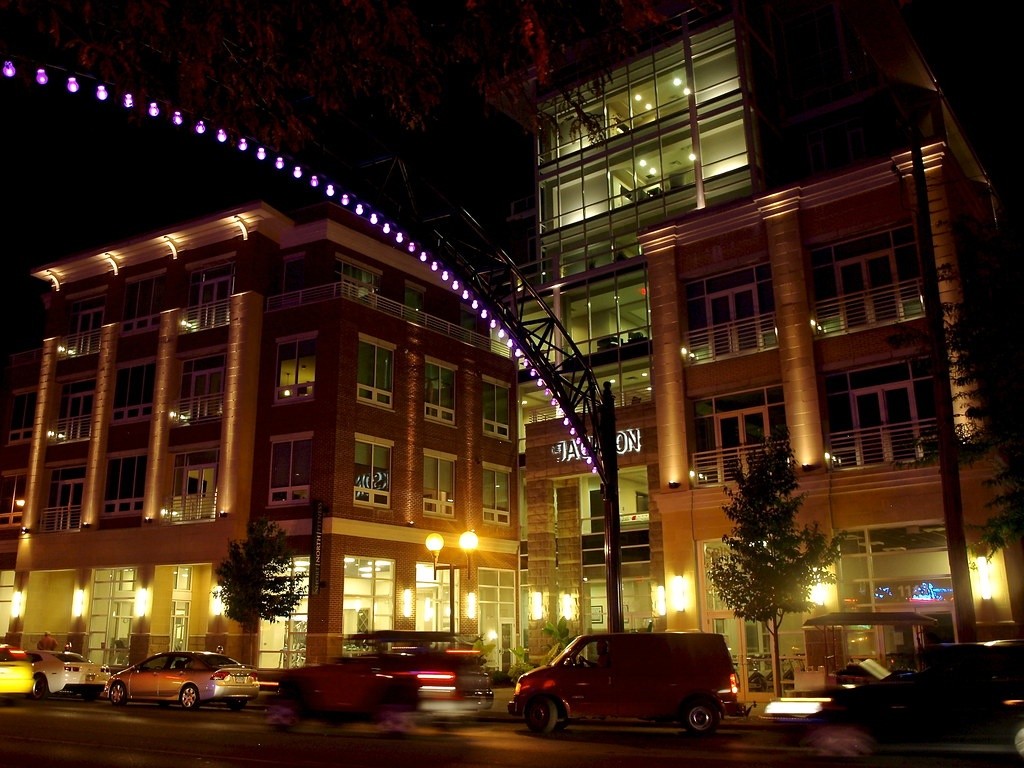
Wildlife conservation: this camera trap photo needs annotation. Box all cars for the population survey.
[21,649,111,703]
[101,648,261,712]
[0,642,33,703]
[262,632,492,738]
[763,640,1023,757]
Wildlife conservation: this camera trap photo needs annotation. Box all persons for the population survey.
[174,660,185,668]
[579,641,608,667]
[37,632,58,650]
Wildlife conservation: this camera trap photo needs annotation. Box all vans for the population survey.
[506,633,738,735]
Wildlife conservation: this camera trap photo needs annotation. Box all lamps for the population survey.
[82,524,91,528]
[218,512,228,518]
[668,482,681,489]
[21,529,30,534]
[144,519,152,523]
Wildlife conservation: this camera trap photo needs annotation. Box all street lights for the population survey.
[425,530,479,650]
